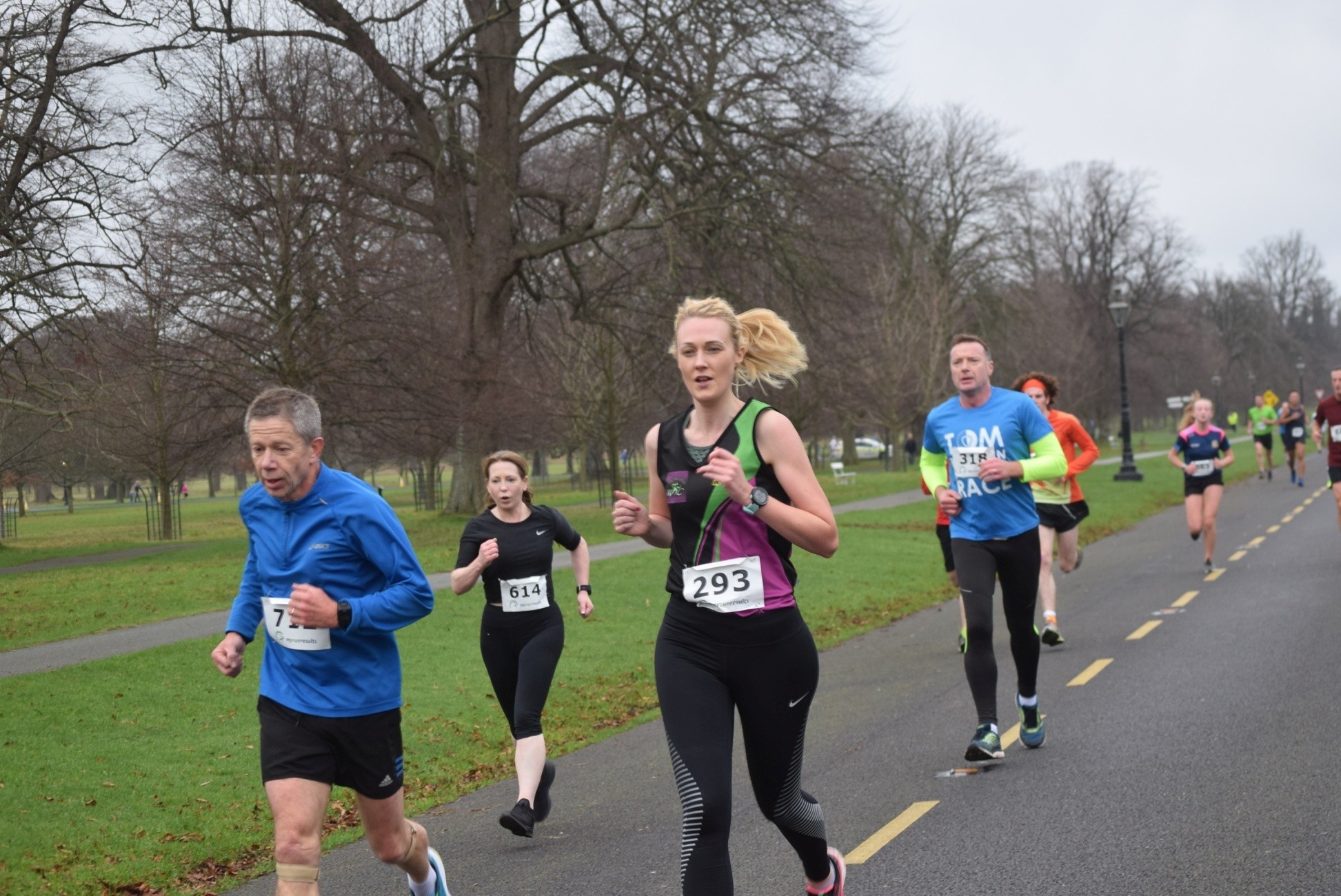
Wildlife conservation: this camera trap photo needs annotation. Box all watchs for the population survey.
[742,486,769,515]
[576,585,592,596]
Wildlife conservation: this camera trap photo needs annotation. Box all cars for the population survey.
[831,438,895,460]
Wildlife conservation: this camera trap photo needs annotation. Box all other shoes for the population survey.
[959,634,965,651]
[1290,473,1296,482]
[1190,533,1199,540]
[1298,478,1303,486]
[1204,560,1216,574]
[1268,471,1271,479]
[1259,472,1264,478]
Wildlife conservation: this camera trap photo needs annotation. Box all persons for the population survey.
[450,450,594,839]
[1226,368,1341,538]
[1167,397,1236,575]
[611,295,847,896]
[918,332,1101,762]
[210,387,452,896]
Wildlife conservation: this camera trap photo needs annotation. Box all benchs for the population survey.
[831,462,857,484]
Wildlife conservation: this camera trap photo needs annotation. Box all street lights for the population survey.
[1294,356,1307,405]
[1105,284,1144,481]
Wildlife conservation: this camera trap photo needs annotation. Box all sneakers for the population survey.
[1041,623,1064,647]
[1015,692,1045,748]
[805,847,846,896]
[500,798,535,838]
[1075,548,1083,568]
[407,846,451,896]
[534,761,555,821]
[965,723,1005,761]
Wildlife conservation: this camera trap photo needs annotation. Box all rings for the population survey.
[993,468,997,474]
[289,608,295,615]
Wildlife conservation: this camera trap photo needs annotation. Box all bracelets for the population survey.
[1181,463,1187,470]
[337,602,352,631]
